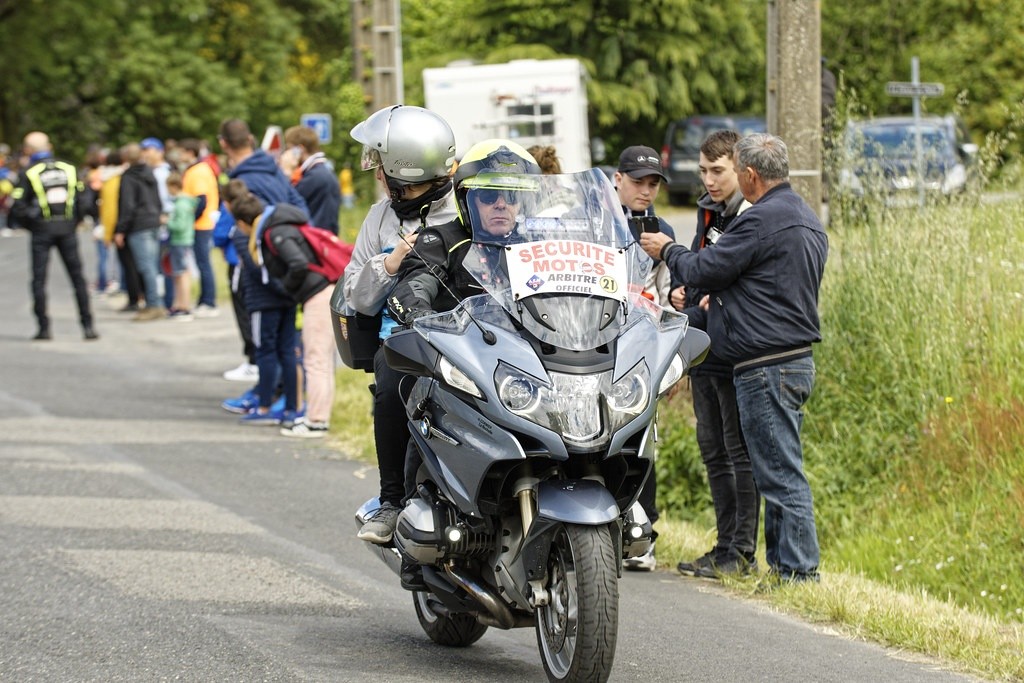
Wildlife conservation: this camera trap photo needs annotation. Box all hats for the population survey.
[617,145,669,183]
[140,137,163,150]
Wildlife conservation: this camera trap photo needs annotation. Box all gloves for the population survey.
[405,308,438,328]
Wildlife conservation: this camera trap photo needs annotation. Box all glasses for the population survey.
[476,189,522,205]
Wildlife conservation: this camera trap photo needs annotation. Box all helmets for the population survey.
[452,138,543,235]
[350,104,456,189]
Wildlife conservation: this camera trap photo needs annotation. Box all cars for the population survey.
[837,115,970,213]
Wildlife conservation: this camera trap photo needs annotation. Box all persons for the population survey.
[0,116,356,427]
[639,132,830,600]
[343,103,459,545]
[228,191,338,440]
[667,128,763,583]
[386,137,629,592]
[524,142,584,220]
[558,144,678,572]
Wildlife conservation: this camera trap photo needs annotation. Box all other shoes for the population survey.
[83,328,99,341]
[133,305,165,321]
[33,327,54,342]
[116,301,140,313]
[193,302,220,318]
[167,308,191,321]
[622,540,657,572]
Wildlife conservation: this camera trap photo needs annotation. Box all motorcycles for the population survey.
[329,169,711,683]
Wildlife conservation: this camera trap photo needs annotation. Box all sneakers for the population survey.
[676,545,759,583]
[356,501,402,542]
[238,401,306,425]
[223,361,260,381]
[279,417,329,437]
[221,391,261,414]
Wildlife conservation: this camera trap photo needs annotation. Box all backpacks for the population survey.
[265,223,355,282]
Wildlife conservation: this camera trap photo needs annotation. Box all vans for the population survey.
[659,116,770,205]
[423,56,608,177]
